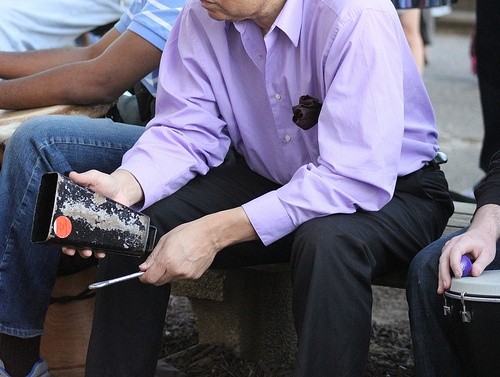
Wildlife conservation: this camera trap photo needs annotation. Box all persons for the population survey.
[391,0,430,79]
[0,1,136,49]
[59,0,456,377]
[0,0,186,377]
[402,0,500,377]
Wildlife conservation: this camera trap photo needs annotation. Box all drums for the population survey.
[0,97,100,377]
[440,269,500,377]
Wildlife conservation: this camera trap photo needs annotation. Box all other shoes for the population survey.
[0,358,51,377]
